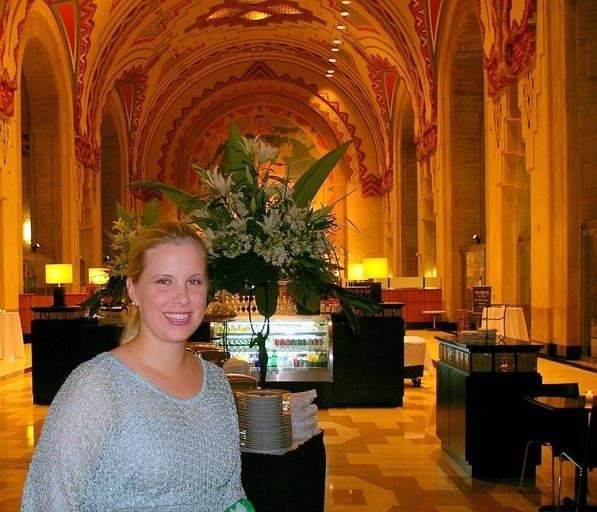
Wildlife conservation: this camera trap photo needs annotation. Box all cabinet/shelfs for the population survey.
[210,314,334,408]
[432,335,545,478]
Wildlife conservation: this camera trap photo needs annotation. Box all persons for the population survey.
[21,219,255,512]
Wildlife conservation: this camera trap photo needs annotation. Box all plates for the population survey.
[235,389,292,451]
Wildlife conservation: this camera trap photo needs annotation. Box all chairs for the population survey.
[518,383,597,512]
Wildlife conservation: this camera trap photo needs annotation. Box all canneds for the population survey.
[273,338,323,347]
[319,299,341,314]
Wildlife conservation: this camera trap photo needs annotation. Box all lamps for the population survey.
[347,264,368,280]
[45,264,73,308]
[88,267,108,286]
[364,258,387,279]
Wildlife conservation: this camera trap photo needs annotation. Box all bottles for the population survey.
[271,349,278,368]
[344,281,370,296]
[277,295,298,314]
[291,352,328,367]
[319,298,341,315]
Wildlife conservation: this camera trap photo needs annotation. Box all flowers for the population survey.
[103,199,160,272]
[127,118,382,390]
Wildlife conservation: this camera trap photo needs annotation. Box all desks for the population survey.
[30,307,85,319]
[240,429,326,512]
[0,312,25,360]
[481,307,529,341]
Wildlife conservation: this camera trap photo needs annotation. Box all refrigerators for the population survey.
[209,316,336,383]
